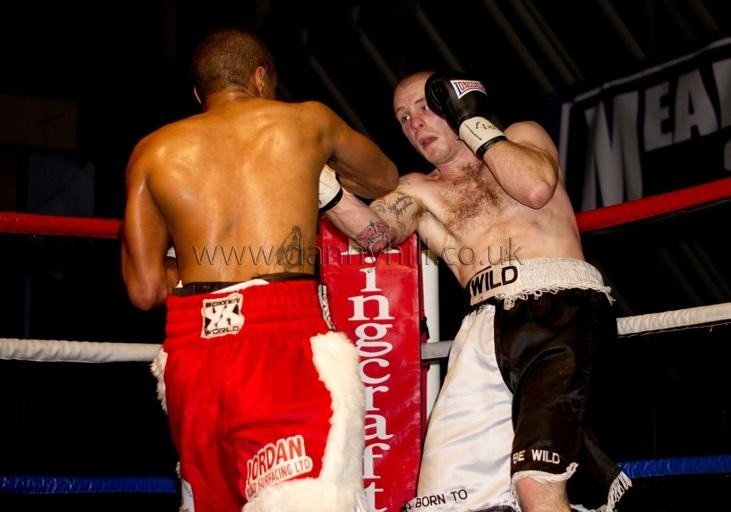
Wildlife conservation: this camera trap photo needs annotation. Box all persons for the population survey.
[318,65,635,511]
[118,26,401,512]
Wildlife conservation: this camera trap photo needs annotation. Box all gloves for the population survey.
[318,165,347,214]
[424,69,510,162]
